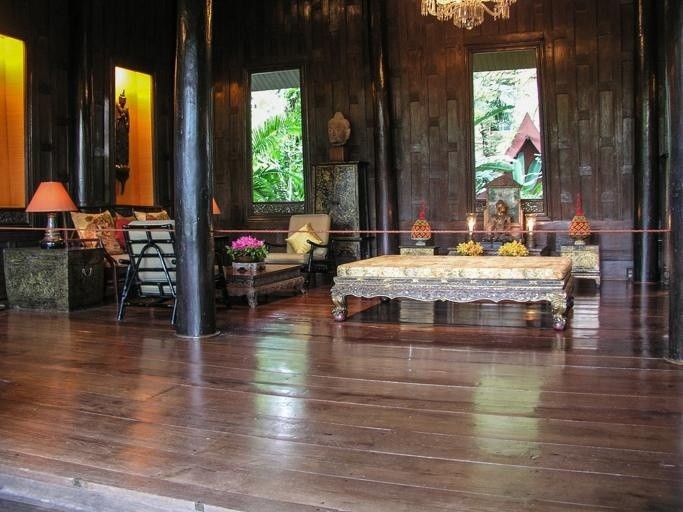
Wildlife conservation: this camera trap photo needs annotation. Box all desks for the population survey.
[559,242,602,288]
[3,242,104,313]
[399,244,439,256]
[330,253,575,332]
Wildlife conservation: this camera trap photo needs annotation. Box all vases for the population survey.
[238,255,255,263]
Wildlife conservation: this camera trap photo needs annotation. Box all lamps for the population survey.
[25,180,78,249]
[420,0,518,34]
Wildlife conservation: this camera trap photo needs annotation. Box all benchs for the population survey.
[69,205,175,317]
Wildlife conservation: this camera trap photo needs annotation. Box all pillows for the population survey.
[71,209,172,258]
[283,222,322,255]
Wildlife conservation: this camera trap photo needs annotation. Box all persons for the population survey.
[115,89,129,165]
[328,112,351,146]
[481,200,514,242]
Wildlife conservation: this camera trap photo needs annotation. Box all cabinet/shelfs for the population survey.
[308,158,373,283]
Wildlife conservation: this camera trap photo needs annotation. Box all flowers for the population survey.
[455,240,529,257]
[225,234,270,261]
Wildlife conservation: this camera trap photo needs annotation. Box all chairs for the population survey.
[266,213,333,288]
[117,219,232,327]
